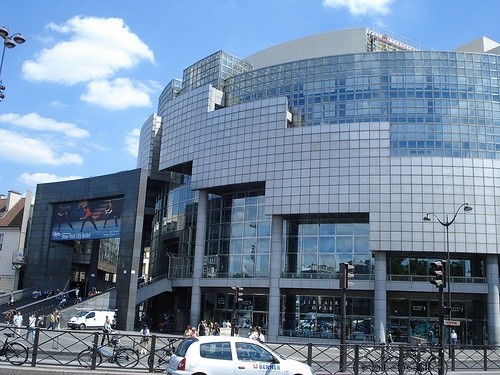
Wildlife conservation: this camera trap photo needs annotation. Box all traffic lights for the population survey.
[346,262,355,289]
[230,286,236,302]
[237,286,244,303]
[251,244,255,253]
[429,259,444,289]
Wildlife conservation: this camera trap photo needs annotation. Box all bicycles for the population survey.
[352,342,448,375]
[147,338,180,373]
[77,338,140,369]
[0,332,30,366]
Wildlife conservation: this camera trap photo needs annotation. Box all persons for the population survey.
[36,309,61,330]
[79,206,98,232]
[248,326,265,358]
[387,325,435,344]
[100,313,152,355]
[159,313,177,334]
[14,310,23,339]
[295,319,374,341]
[32,284,102,309]
[26,311,36,340]
[57,207,74,231]
[103,204,120,228]
[4,291,18,325]
[387,331,393,351]
[183,313,249,338]
[451,330,457,348]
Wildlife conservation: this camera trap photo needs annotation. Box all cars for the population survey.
[164,335,317,375]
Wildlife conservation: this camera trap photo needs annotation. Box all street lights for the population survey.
[250,222,257,278]
[422,203,473,358]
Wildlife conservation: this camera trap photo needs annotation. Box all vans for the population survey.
[67,310,116,330]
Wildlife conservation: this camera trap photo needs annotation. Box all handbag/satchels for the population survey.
[27,325,29,328]
[388,339,391,343]
